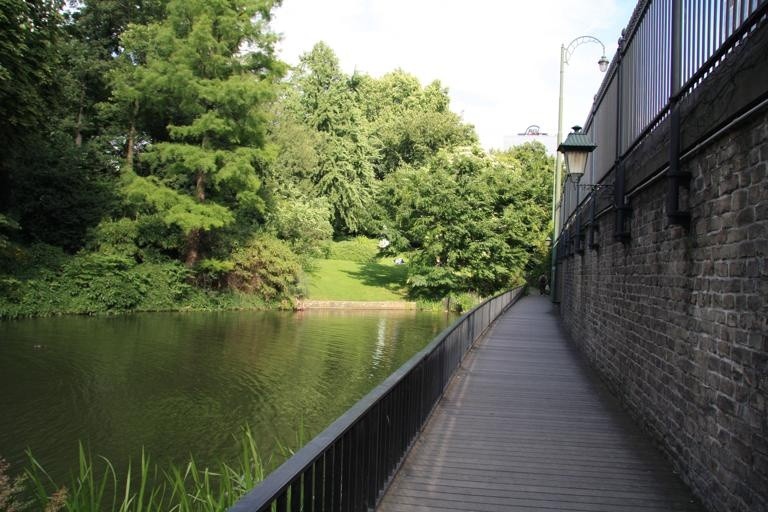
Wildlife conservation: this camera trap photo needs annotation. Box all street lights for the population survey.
[552,34,609,298]
